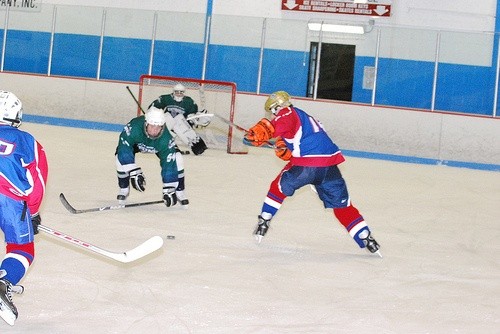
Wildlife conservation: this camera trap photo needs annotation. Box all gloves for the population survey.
[242,118,275,148]
[274,137,291,160]
[161,182,179,207]
[129,167,146,192]
[30,211,42,235]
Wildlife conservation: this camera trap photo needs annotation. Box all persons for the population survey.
[243,92,382,258]
[114,106,190,209]
[148,83,214,155]
[0,89,48,327]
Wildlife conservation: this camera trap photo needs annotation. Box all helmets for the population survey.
[263,90,291,113]
[0,89,23,127]
[172,84,185,102]
[145,106,167,127]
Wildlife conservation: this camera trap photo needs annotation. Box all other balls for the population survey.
[166,235,176,240]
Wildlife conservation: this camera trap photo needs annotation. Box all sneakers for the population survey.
[175,191,189,209]
[116,184,130,205]
[0,268,24,326]
[364,235,384,258]
[252,215,272,242]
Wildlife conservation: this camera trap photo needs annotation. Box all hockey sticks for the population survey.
[59,192,166,215]
[35,224,164,263]
[126,85,145,115]
[187,112,283,150]
